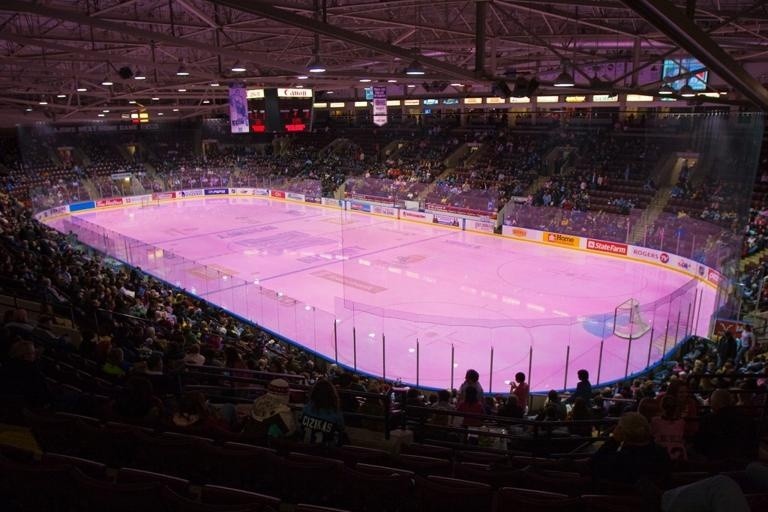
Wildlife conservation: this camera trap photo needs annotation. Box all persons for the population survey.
[0,107,768,510]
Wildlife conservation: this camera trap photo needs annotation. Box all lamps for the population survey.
[405,2,424,75]
[553,5,575,87]
[657,57,672,95]
[680,56,695,98]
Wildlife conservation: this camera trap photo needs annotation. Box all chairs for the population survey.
[585,149,768,258]
